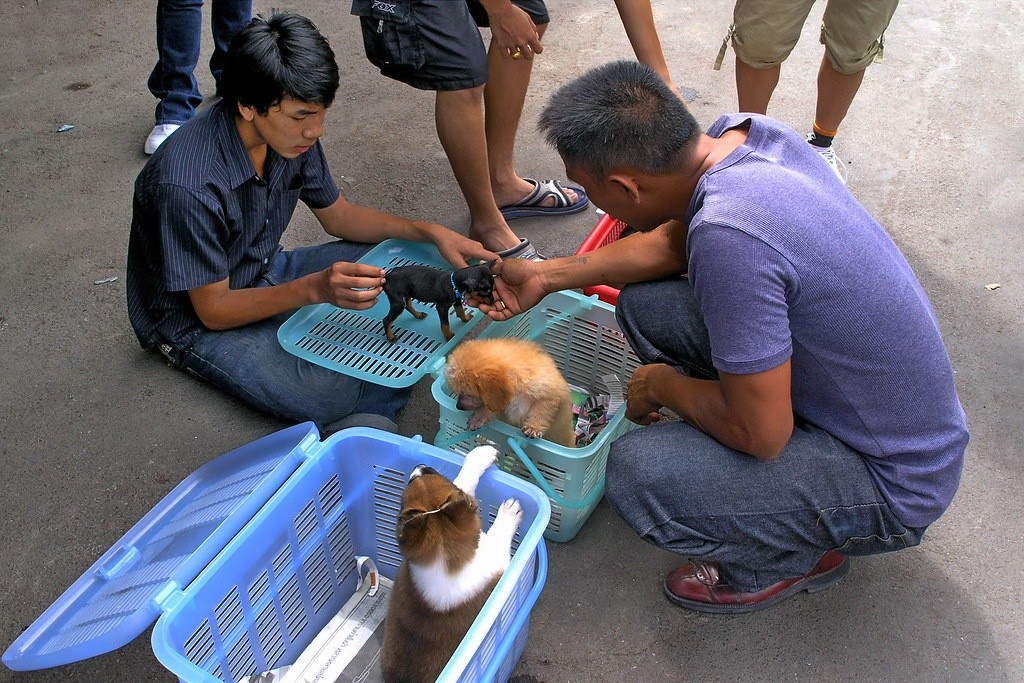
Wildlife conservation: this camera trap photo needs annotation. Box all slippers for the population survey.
[493,238,548,262]
[499,178,590,221]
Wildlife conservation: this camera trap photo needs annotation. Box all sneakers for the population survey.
[806,131,846,185]
[145,124,181,154]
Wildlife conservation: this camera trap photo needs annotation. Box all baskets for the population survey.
[574,212,630,307]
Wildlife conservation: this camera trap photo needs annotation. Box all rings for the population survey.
[511,51,523,61]
[500,299,505,309]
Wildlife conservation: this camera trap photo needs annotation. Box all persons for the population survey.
[126,7,503,440]
[350,0,687,261]
[713,0,899,185]
[144,0,252,157]
[464,58,971,615]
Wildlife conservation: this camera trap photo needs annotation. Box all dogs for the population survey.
[378,445,525,683]
[382,259,498,344]
[444,338,575,484]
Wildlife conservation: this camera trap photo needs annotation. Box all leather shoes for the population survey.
[663,547,850,614]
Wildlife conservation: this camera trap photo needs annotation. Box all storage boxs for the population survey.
[2,420,551,683]
[276,237,645,543]
[575,214,621,305]
